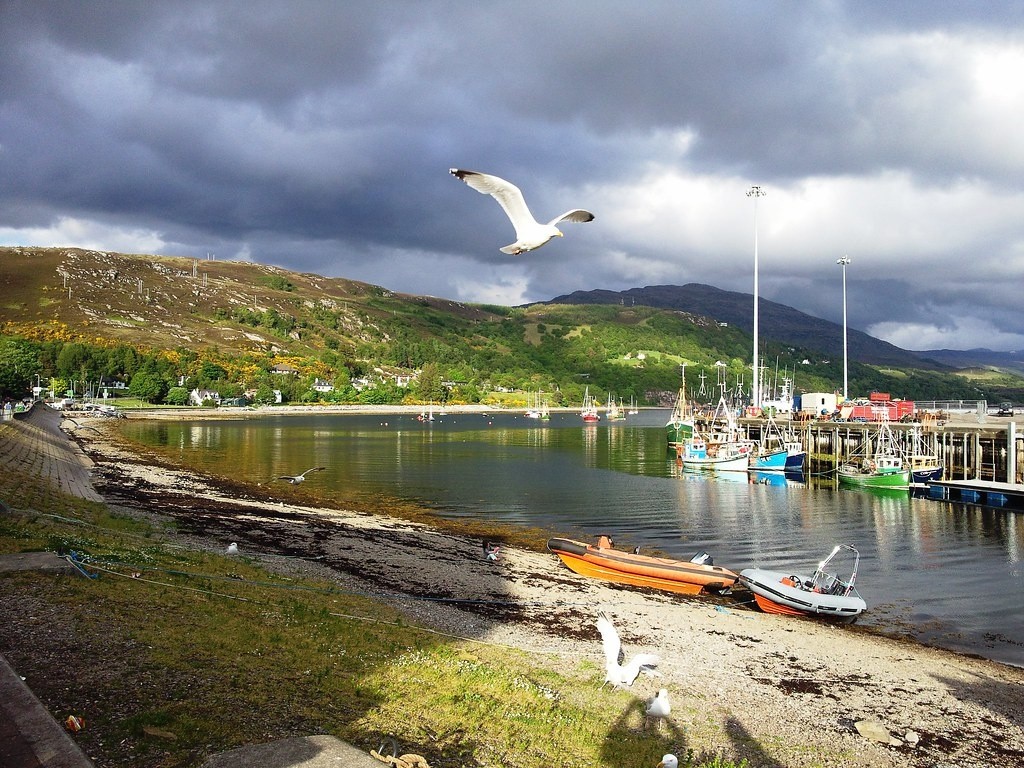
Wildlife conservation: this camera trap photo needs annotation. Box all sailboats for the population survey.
[665,361,943,493]
[526,386,551,420]
[418,396,448,423]
[580,386,640,422]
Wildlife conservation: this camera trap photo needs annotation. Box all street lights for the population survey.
[747,185,767,406]
[34,374,39,399]
[51,377,55,402]
[837,256,852,398]
[69,380,94,405]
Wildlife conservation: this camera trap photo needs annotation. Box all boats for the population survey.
[739,542,867,622]
[546,534,738,599]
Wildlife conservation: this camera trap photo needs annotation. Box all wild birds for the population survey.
[447,166,596,258]
[67,418,104,437]
[595,609,659,693]
[263,466,326,487]
[640,687,673,732]
[654,752,680,768]
[224,541,242,556]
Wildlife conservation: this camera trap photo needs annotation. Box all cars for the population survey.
[999,402,1014,417]
